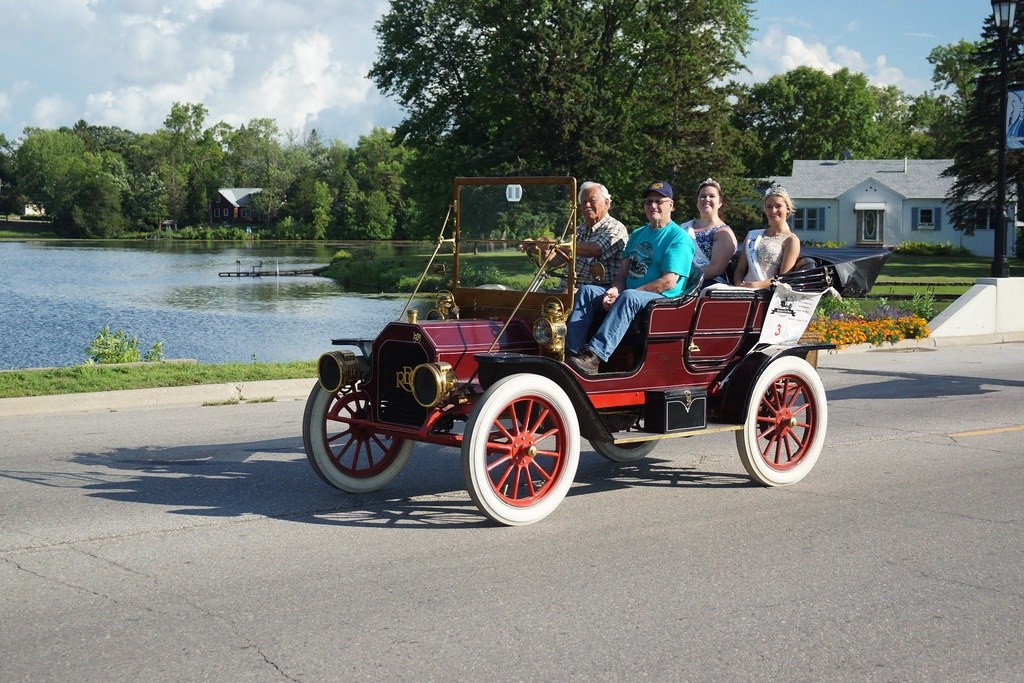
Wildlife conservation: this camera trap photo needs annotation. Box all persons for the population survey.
[731,186,800,288]
[567,180,697,374]
[679,180,739,291]
[520,181,630,293]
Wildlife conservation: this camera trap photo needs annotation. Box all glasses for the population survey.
[643,199,671,205]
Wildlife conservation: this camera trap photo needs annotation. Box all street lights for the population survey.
[990,0,1018,278]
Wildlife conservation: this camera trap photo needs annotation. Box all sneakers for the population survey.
[569,351,599,374]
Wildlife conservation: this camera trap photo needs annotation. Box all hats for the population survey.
[643,180,674,198]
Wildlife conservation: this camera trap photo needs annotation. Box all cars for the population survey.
[300,176,894,527]
